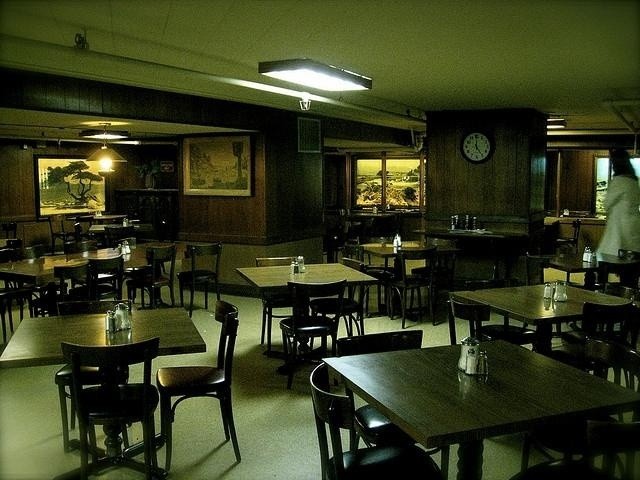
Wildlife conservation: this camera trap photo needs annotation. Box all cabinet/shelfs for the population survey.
[113,188,179,240]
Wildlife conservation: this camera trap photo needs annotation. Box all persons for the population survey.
[596,149,640,257]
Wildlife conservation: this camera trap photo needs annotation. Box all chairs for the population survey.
[158,300,241,472]
[60,336,159,478]
[334,327,450,479]
[310,362,449,480]
[53,298,131,449]
[279,278,347,392]
[2,211,639,340]
[549,300,635,387]
[511,419,638,478]
[521,338,638,467]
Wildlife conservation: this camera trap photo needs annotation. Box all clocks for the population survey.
[461,131,495,164]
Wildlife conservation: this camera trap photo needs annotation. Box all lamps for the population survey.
[86,124,129,173]
[79,129,131,141]
[259,57,372,93]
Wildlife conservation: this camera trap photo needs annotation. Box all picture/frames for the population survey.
[180,132,255,197]
[34,153,110,219]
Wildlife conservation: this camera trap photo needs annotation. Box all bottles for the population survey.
[544,298,551,311]
[583,246,601,266]
[118,238,131,255]
[393,234,401,247]
[123,218,128,227]
[543,280,568,302]
[105,302,132,333]
[290,256,306,273]
[457,336,490,377]
[449,214,478,229]
